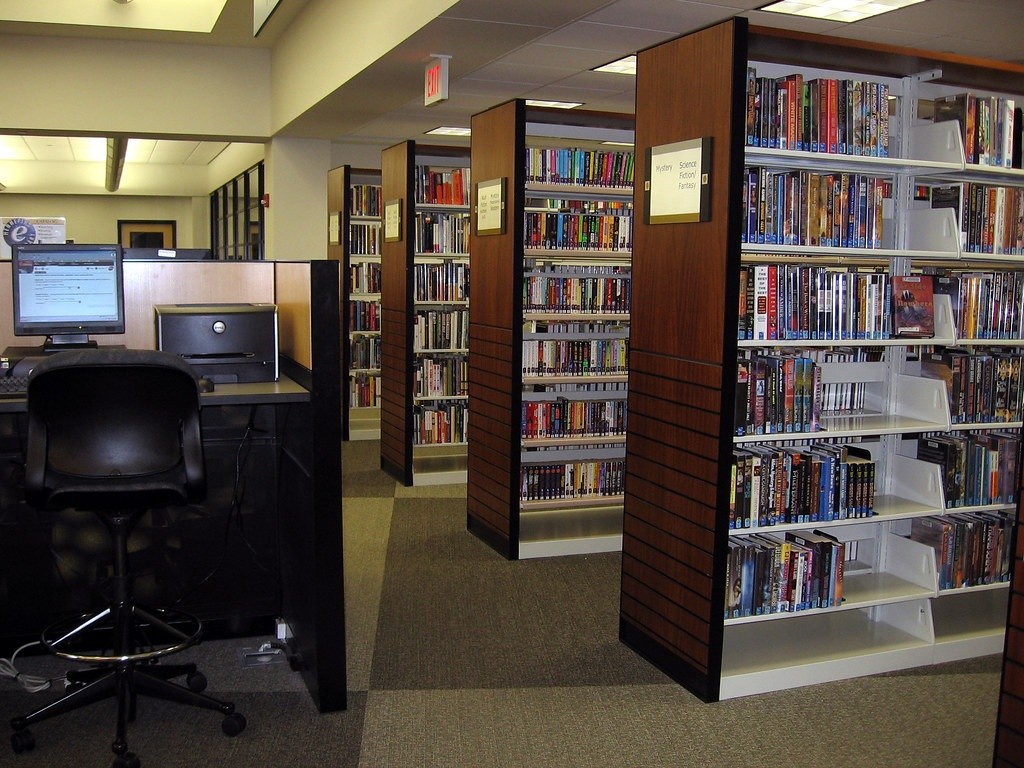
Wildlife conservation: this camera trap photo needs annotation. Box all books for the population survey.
[718,64,1024,621]
[410,164,472,448]
[514,146,637,504]
[346,183,384,411]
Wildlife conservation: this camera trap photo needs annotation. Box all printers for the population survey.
[154,302,279,383]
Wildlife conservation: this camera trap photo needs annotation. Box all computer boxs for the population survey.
[0,344,127,377]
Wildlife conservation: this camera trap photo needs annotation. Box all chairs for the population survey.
[5,345,247,768]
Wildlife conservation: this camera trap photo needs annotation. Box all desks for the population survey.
[1,255,349,717]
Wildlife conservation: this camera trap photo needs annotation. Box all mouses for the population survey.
[197,378,214,393]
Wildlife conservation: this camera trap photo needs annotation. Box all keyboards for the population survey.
[0,376,28,399]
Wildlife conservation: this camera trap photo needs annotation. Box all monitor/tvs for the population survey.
[122,248,212,259]
[11,243,126,351]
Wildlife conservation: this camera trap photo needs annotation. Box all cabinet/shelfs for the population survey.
[322,12,1024,768]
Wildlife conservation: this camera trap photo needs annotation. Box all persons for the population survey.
[732,578,742,603]
